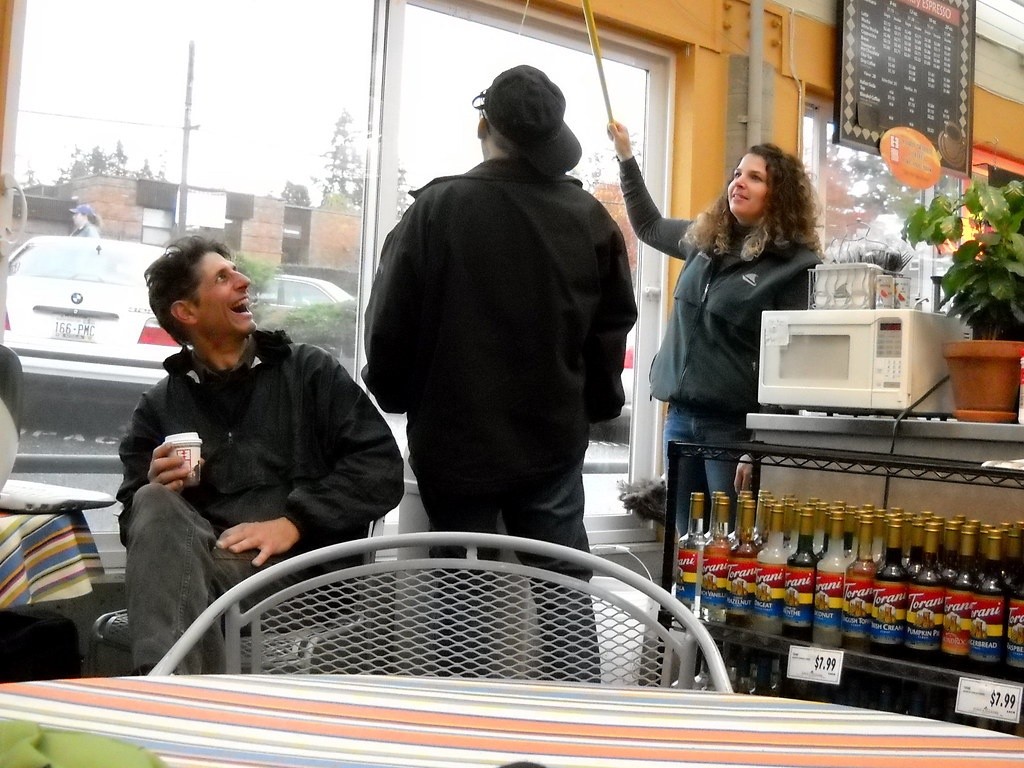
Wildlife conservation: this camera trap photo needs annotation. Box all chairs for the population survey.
[147,525,732,693]
[85,510,377,674]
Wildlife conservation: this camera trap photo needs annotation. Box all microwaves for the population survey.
[757,308,972,418]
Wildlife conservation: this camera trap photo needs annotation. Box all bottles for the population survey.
[674,487,1024,738]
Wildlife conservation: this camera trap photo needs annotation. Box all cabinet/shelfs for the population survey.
[659,436,1024,724]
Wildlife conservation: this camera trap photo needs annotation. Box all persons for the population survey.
[115,235,404,677]
[362,66,639,683]
[608,119,824,538]
[69,204,102,237]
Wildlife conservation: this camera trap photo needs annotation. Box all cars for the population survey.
[0,235,192,351]
[253,273,358,314]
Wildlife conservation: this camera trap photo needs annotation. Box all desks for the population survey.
[0,665,1024,768]
[0,511,107,609]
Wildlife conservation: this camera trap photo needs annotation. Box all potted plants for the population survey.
[901,167,1024,422]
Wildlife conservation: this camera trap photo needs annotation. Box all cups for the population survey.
[895,278,911,308]
[875,275,894,309]
[163,432,204,487]
[944,121,967,158]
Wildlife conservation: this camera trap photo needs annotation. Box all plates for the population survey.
[937,130,966,167]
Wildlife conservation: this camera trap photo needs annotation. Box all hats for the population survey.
[486,65,583,176]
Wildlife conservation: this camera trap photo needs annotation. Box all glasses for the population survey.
[472,90,490,134]
